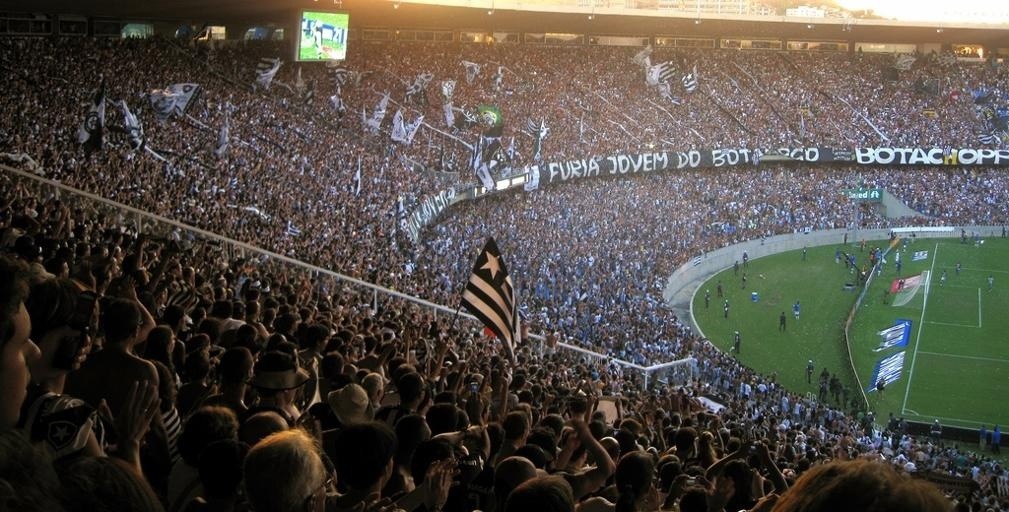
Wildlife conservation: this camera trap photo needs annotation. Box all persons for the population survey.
[0,34,1009,510]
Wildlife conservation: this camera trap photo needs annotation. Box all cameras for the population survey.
[748,443,762,456]
[684,476,701,489]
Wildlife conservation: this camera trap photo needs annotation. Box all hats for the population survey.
[495,455,549,496]
[250,350,310,389]
[328,384,374,427]
